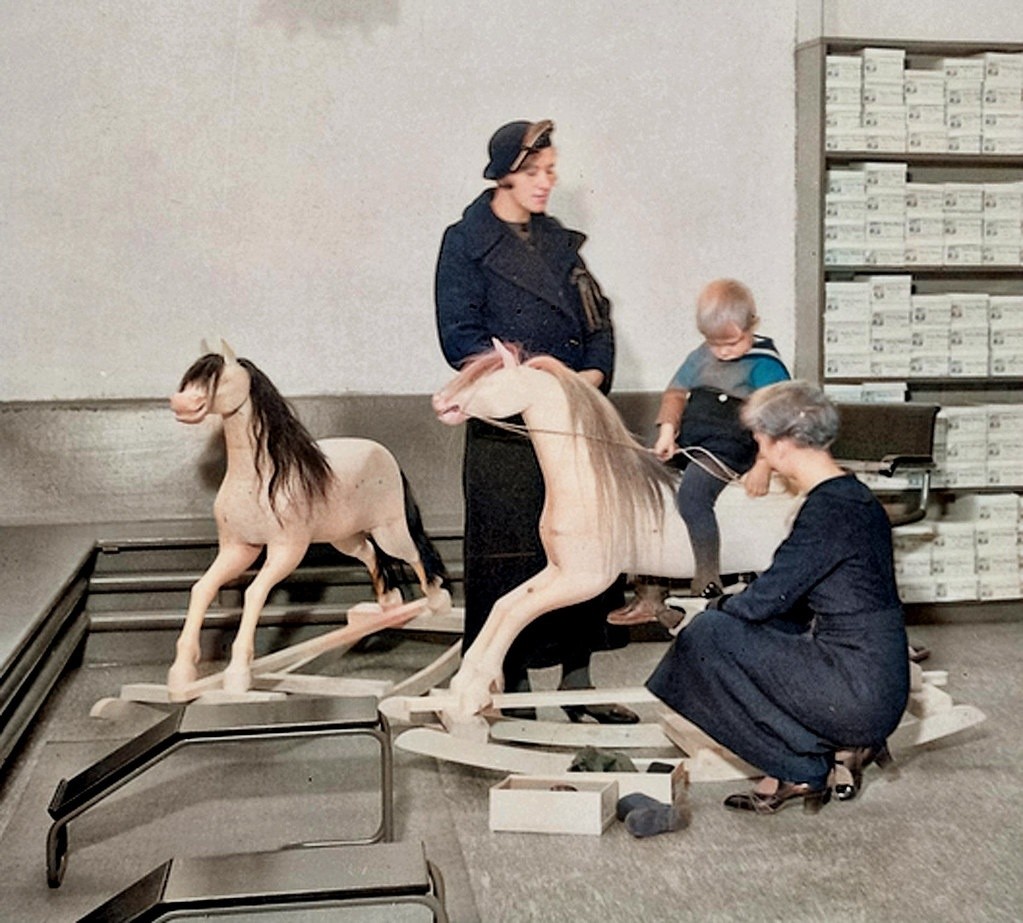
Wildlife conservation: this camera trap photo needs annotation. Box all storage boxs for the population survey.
[487,775,621,838]
[558,760,687,809]
[824,48,1021,602]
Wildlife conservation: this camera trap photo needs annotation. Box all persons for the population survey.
[643,378,911,816]
[608,278,792,625]
[434,121,644,725]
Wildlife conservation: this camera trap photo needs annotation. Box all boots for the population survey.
[613,792,690,837]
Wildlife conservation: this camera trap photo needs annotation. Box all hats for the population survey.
[483,121,554,180]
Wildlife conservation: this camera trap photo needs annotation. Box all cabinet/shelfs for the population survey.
[794,34,1022,609]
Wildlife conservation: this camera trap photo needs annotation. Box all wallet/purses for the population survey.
[564,269,605,334]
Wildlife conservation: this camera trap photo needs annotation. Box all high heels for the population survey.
[557,687,639,725]
[725,772,827,816]
[829,735,893,800]
[500,673,536,719]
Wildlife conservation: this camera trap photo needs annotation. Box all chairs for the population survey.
[830,402,943,661]
